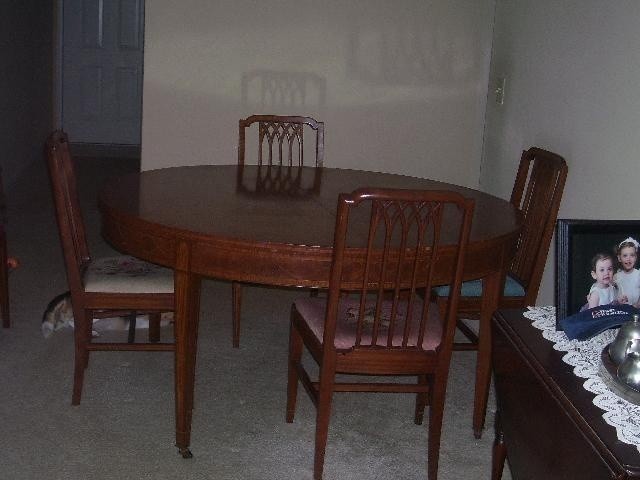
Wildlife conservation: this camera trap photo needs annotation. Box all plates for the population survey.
[599,342,640,409]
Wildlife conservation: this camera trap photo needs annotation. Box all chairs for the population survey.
[285,188,477,479]
[47,129,195,404]
[231,115,326,349]
[412,145,570,439]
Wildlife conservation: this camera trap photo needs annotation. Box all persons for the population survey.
[586,237,640,310]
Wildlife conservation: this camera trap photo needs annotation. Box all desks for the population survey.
[102,166,524,477]
[488,304,640,479]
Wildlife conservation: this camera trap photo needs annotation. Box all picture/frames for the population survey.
[552,217,640,332]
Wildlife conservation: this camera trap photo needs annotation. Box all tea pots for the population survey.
[611,316,640,392]
